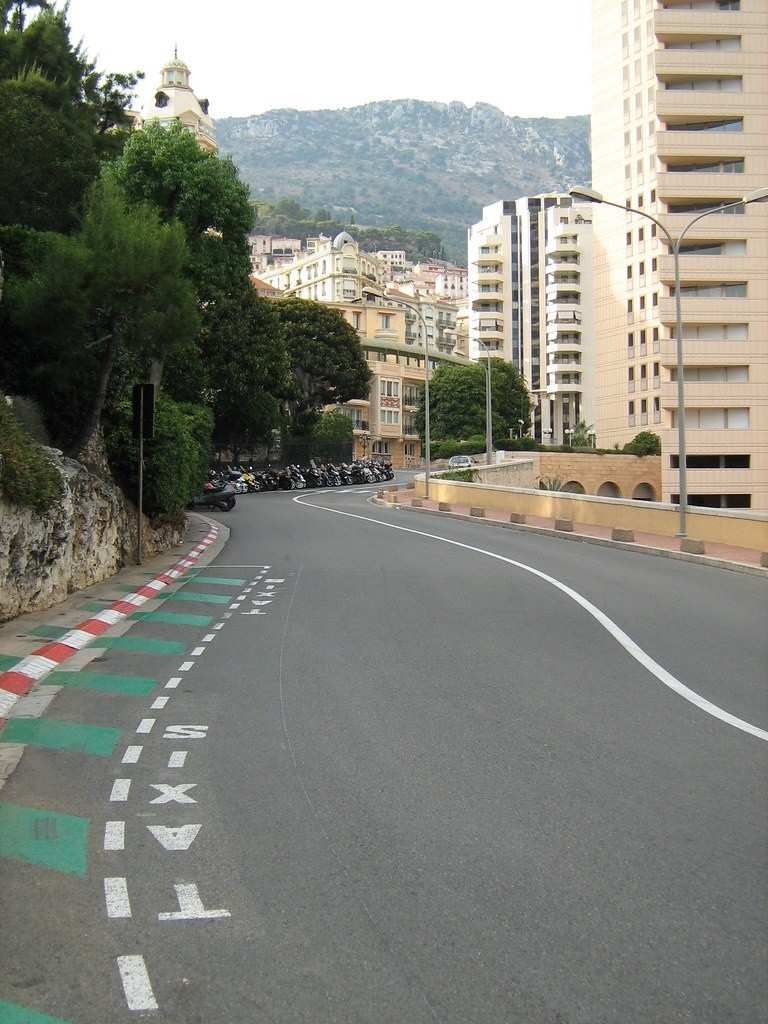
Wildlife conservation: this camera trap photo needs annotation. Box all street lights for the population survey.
[588,429,595,448]
[565,429,574,447]
[568,186,768,538]
[363,286,431,499]
[444,328,492,464]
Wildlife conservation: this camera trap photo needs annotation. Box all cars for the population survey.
[447,456,480,470]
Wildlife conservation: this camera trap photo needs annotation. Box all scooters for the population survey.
[187,455,395,511]
